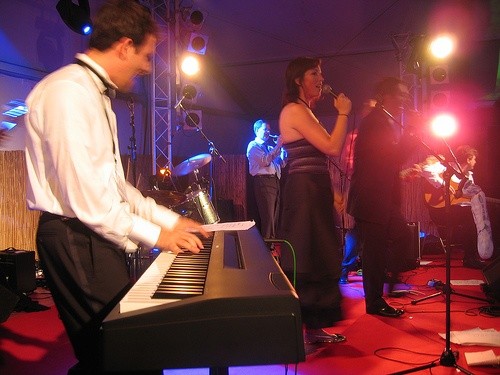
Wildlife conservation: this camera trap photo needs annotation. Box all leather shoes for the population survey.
[366,302,404,316]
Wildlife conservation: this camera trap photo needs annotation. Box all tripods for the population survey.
[380,108,493,375]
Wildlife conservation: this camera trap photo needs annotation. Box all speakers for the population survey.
[0,249,36,293]
[480,253,500,292]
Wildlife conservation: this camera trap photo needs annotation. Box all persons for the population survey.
[442,146,486,269]
[24,1,208,375]
[345,75,424,319]
[277,56,352,344]
[247,119,288,253]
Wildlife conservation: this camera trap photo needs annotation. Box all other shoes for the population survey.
[304,333,346,344]
[464,258,487,270]
[340,276,349,283]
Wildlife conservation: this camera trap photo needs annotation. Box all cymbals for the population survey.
[142,189,187,206]
[173,153,211,177]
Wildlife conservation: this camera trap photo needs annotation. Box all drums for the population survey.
[171,188,221,225]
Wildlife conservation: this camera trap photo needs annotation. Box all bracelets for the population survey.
[338,113,350,118]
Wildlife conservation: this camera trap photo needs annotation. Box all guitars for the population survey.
[424,173,500,210]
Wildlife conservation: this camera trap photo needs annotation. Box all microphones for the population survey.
[323,84,337,98]
[174,92,188,109]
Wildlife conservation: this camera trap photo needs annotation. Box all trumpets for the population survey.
[268,134,279,144]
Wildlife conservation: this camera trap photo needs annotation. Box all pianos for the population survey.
[98,218,307,375]
